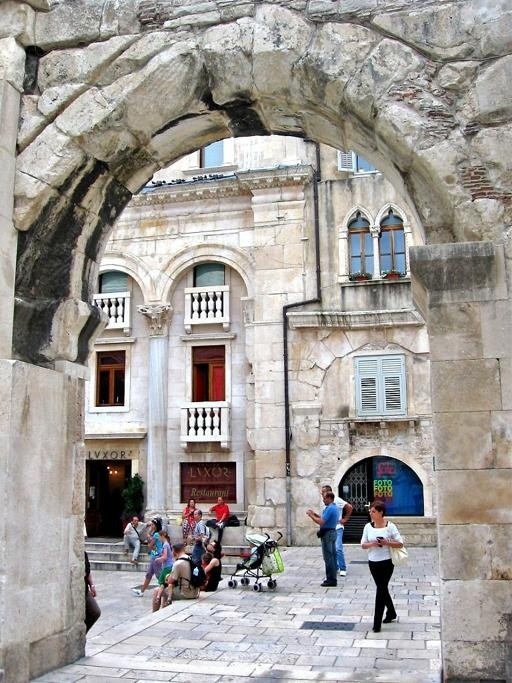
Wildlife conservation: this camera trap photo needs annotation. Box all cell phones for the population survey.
[376,537,384,541]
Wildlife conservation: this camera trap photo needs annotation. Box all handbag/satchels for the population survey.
[317,528,331,538]
[262,547,284,574]
[229,514,240,526]
[389,538,408,565]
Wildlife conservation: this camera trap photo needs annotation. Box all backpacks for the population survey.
[205,525,212,544]
[176,556,206,587]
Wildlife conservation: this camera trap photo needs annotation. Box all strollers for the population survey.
[228,530,283,592]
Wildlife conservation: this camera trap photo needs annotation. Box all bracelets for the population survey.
[387,540,392,546]
[89,584,95,588]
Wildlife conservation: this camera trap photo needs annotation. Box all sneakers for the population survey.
[133,589,144,597]
[321,581,337,586]
[340,571,346,576]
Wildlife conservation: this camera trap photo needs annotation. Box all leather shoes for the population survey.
[374,628,380,632]
[383,616,396,623]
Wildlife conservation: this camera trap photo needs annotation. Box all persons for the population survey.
[247,542,258,567]
[359,499,405,632]
[123,496,230,607]
[320,485,353,577]
[306,491,340,588]
[84,550,102,635]
